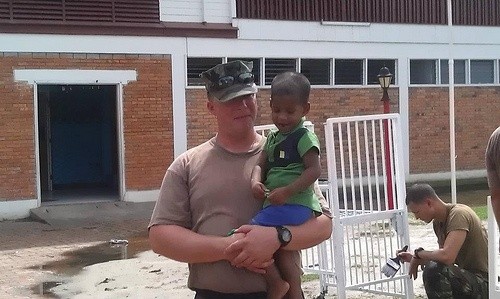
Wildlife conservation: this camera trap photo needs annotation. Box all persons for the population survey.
[486,126,500,253]
[243,72,322,299]
[396,183,488,299]
[148,60,333,299]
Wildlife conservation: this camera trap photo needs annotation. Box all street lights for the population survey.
[376,66,393,210]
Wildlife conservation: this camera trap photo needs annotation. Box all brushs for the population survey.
[380,245,409,279]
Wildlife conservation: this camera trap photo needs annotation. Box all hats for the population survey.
[199,61,258,102]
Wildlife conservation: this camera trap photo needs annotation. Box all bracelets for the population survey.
[414,247,424,259]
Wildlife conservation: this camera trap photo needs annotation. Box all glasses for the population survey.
[218,72,253,88]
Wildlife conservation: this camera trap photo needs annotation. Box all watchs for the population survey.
[275,225,292,248]
